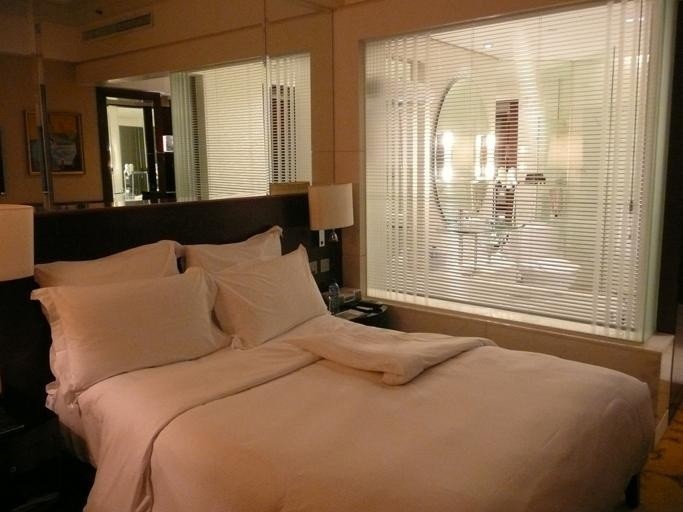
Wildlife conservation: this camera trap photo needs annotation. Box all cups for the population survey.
[353,289,361,302]
[162,135,174,152]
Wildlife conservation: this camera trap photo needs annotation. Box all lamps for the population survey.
[440,130,452,182]
[473,134,482,177]
[484,134,496,179]
[0,203,35,284]
[308,182,353,308]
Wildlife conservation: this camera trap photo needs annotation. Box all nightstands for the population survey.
[330,301,392,327]
[0,390,65,512]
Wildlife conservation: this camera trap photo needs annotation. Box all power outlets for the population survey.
[309,258,329,275]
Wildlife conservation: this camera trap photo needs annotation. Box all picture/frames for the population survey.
[25,110,85,175]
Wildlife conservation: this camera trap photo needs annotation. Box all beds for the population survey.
[32,193,652,512]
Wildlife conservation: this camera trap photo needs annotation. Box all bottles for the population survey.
[498,167,516,183]
[124,162,134,202]
[329,278,340,314]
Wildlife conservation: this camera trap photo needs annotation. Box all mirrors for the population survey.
[433,77,493,228]
[0,0,335,212]
